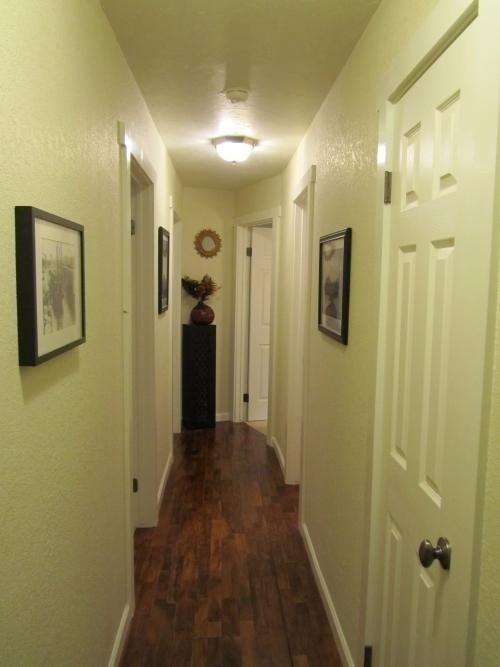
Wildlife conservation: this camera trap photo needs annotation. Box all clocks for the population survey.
[192,228,222,258]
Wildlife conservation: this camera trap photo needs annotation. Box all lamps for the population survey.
[211,135,258,166]
[181,273,221,325]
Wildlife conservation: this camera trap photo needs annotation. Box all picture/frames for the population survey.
[158,226,169,313]
[15,205,86,366]
[318,227,352,345]
